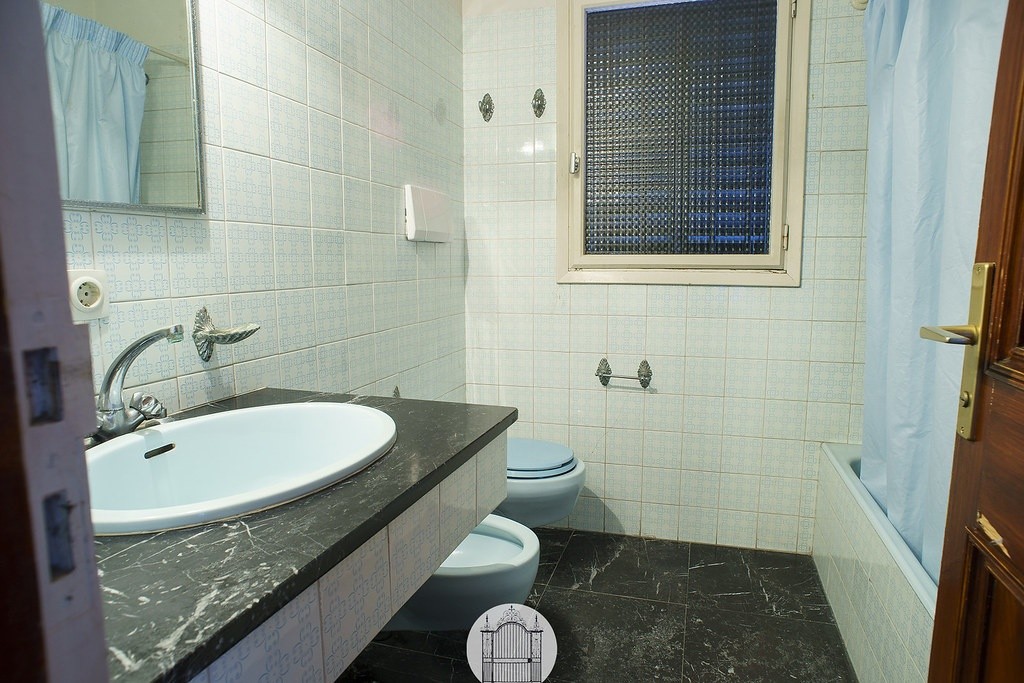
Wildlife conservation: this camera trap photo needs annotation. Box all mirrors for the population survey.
[38,0,210,216]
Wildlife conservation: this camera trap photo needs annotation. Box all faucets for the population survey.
[76,321,185,447]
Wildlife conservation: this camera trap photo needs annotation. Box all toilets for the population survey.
[494,436,587,528]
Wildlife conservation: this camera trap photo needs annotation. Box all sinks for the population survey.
[384,510,540,632]
[86,402,398,537]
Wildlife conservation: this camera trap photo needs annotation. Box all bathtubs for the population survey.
[808,441,938,683]
